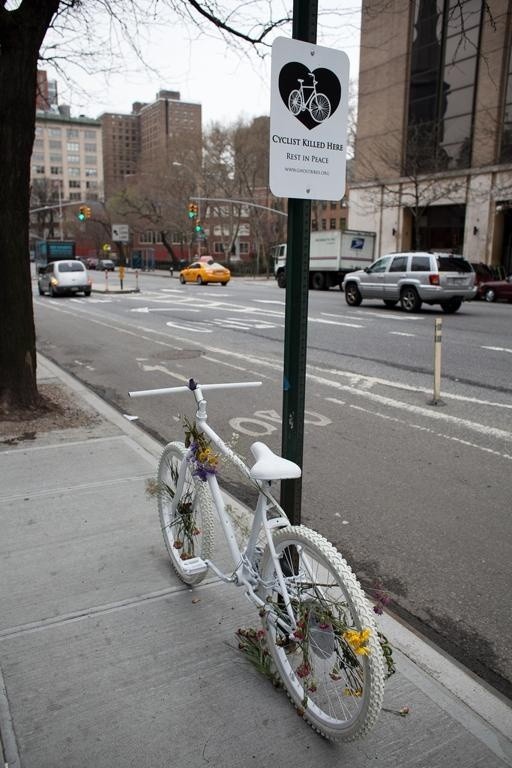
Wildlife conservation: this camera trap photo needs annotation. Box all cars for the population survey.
[75,256,115,272]
[179,261,230,286]
[38,260,92,298]
[471,262,512,303]
[28,250,36,261]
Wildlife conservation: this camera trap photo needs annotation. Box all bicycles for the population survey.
[127,378,386,744]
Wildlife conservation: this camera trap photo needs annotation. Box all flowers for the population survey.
[145,417,224,560]
[222,576,409,717]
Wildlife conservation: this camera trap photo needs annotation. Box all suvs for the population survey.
[341,251,478,314]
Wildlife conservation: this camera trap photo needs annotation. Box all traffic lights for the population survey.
[78,205,91,222]
[188,203,198,219]
[192,219,206,241]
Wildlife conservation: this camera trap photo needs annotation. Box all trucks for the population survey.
[274,230,377,290]
[35,240,76,274]
